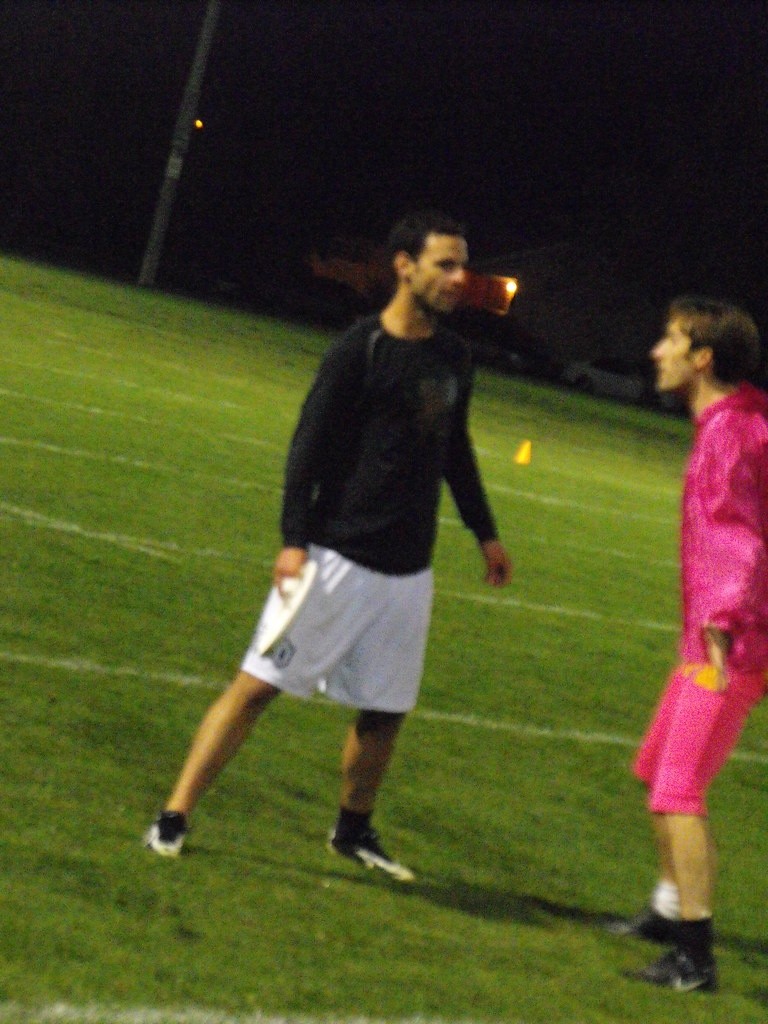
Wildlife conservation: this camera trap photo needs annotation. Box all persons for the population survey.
[603,296,768,993]
[143,206,514,884]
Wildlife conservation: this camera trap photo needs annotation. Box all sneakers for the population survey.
[326,829,416,884]
[149,812,190,857]
[625,944,718,993]
[606,908,680,945]
